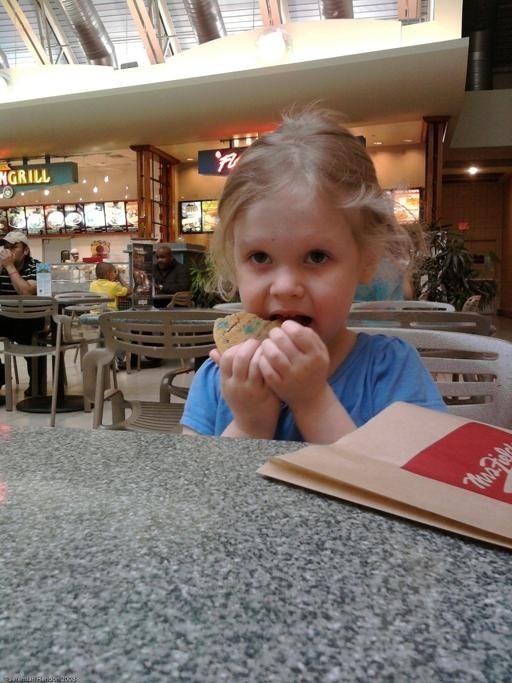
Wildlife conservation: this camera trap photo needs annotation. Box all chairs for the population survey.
[1,292,511,432]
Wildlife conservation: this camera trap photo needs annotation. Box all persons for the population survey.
[86,261,134,372]
[177,98,449,446]
[350,250,414,328]
[146,245,191,308]
[0,231,47,397]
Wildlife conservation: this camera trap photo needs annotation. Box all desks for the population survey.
[0,415,512,682]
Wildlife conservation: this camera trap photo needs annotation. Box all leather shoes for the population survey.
[24,387,32,395]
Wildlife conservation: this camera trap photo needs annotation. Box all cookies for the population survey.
[210,312,288,361]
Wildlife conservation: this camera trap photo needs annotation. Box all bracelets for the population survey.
[9,272,18,275]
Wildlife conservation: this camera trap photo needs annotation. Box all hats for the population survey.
[0,231,29,248]
